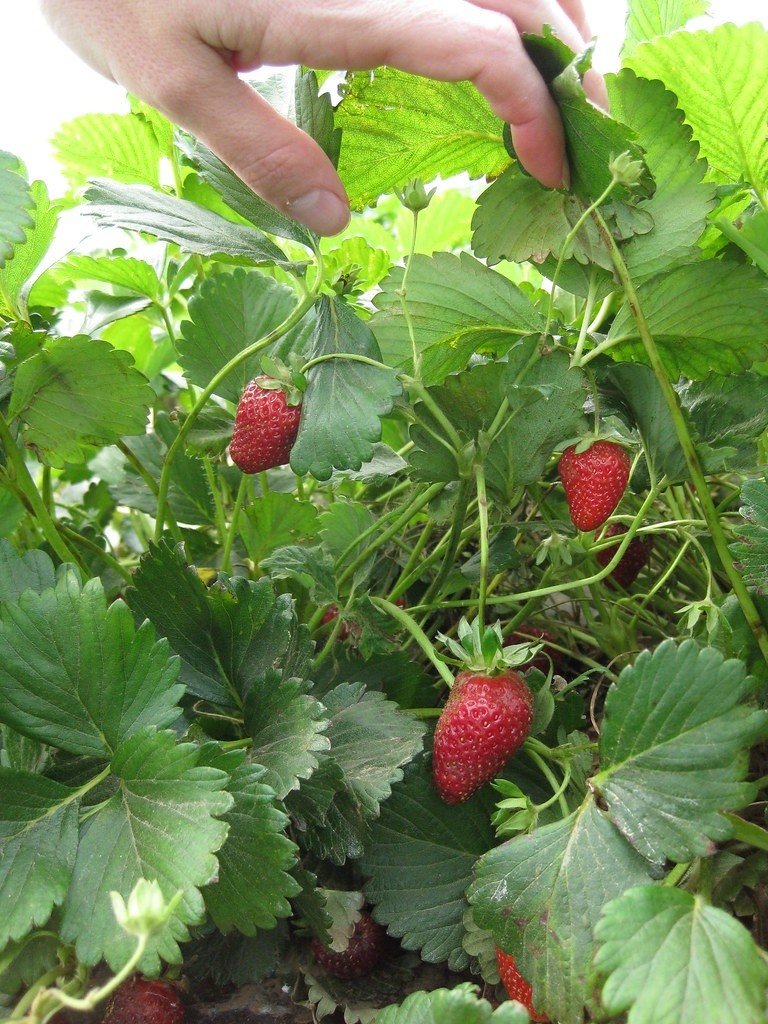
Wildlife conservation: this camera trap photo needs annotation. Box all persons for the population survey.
[37,1,613,238]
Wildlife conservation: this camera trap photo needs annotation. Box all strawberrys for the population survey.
[229,372,304,474]
[315,594,408,662]
[500,625,558,678]
[310,905,381,982]
[495,943,552,1024]
[554,432,642,532]
[591,521,654,592]
[431,617,535,804]
[13,978,185,1024]
